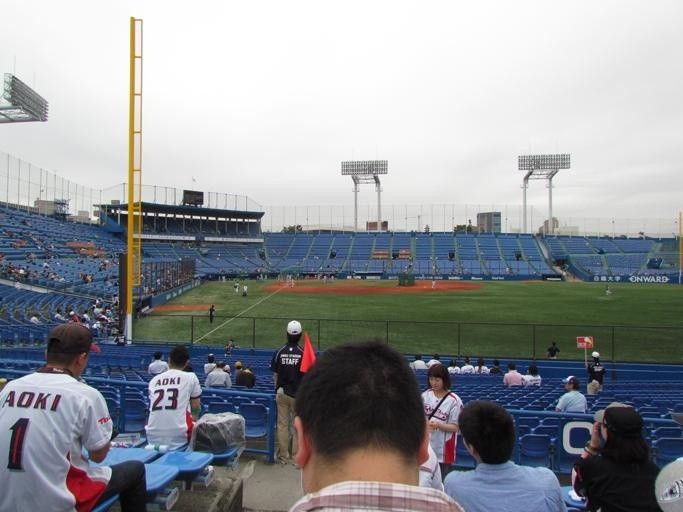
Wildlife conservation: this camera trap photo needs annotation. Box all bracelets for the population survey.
[583,447,593,458]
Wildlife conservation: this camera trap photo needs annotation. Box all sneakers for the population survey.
[276,450,287,465]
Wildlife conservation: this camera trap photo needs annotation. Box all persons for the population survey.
[413,354,426,369]
[141,257,195,294]
[144,345,200,446]
[589,350,605,393]
[234,360,242,375]
[208,305,214,324]
[475,358,489,374]
[205,360,232,387]
[489,359,503,374]
[522,363,541,387]
[55,298,119,334]
[554,374,587,413]
[290,340,463,511]
[0,215,123,259]
[446,360,461,374]
[445,399,566,511]
[223,364,232,374]
[546,340,559,358]
[4,337,14,345]
[0,324,144,512]
[225,339,235,354]
[420,446,445,487]
[571,400,660,511]
[34,338,42,346]
[502,363,522,386]
[419,363,463,478]
[426,354,441,368]
[0,259,122,290]
[272,321,306,468]
[203,355,216,375]
[19,338,28,347]
[461,357,476,374]
[148,350,169,373]
[243,285,248,296]
[236,366,256,387]
[234,281,240,293]
[286,273,292,283]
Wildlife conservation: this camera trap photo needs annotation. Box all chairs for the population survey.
[0,207,155,299]
[403,352,683,512]
[128,216,271,296]
[80,343,282,512]
[543,233,683,283]
[264,232,562,282]
[0,344,47,382]
[0,285,116,344]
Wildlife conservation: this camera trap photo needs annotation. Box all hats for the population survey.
[287,320,302,335]
[593,402,641,429]
[562,375,576,384]
[591,351,600,358]
[47,322,100,354]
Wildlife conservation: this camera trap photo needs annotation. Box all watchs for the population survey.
[585,440,600,452]
